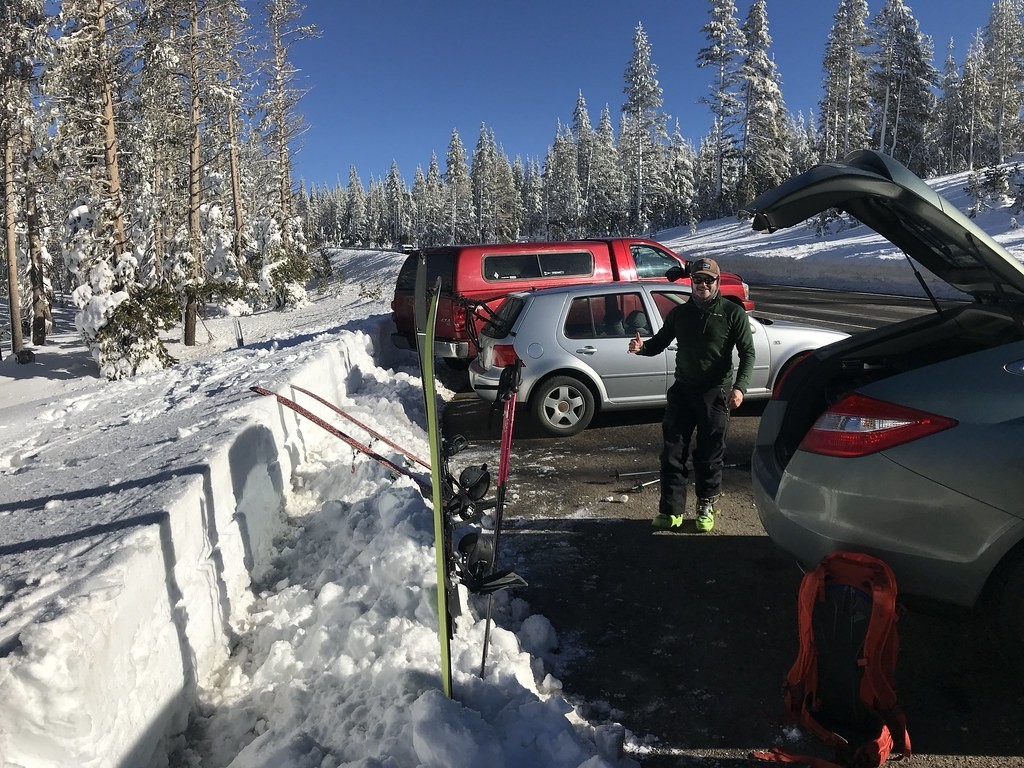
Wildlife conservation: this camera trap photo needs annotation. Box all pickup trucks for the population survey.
[391,238,757,376]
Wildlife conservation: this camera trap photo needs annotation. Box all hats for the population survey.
[691,258,720,280]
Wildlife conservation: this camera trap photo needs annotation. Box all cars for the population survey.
[738,148,1024,700]
[467,280,851,436]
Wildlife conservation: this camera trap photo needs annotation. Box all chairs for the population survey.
[625,311,650,336]
[603,309,624,336]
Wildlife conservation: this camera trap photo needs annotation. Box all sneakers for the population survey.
[651,513,684,528]
[695,496,716,532]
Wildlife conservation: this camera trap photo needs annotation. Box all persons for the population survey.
[625,258,755,532]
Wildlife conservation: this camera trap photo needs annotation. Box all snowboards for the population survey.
[422,273,455,702]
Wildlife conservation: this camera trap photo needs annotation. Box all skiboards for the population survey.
[248,382,475,505]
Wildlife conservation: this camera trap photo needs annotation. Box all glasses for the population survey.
[692,276,717,284]
